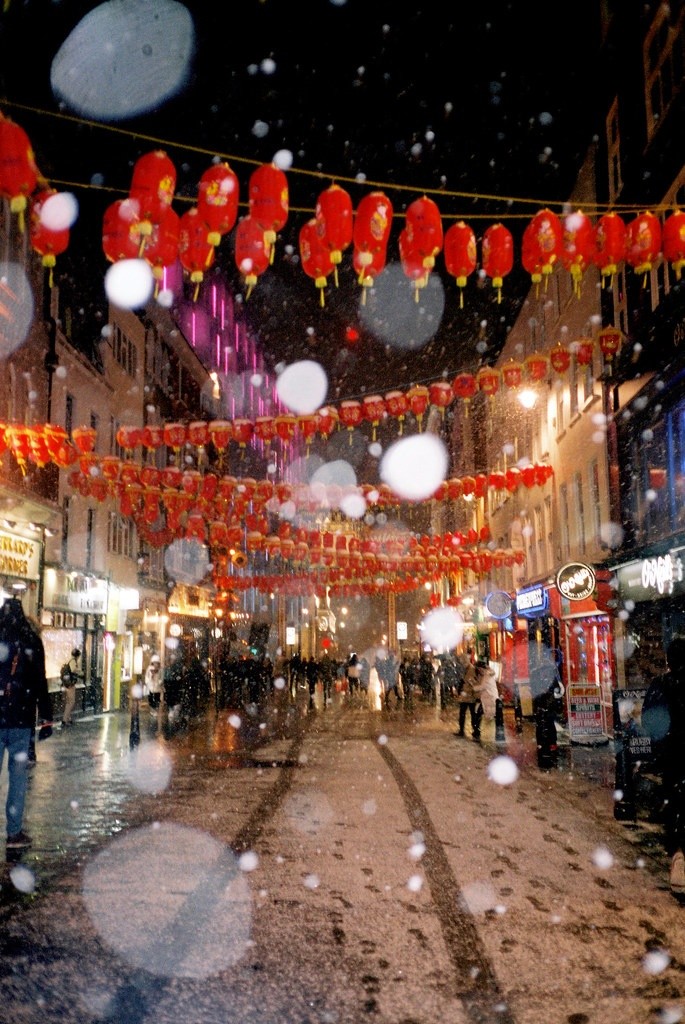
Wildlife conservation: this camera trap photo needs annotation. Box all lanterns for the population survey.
[0,424,97,476]
[120,501,527,604]
[299,185,514,306]
[117,327,620,468]
[67,453,554,522]
[102,151,289,303]
[0,112,71,288]
[522,210,685,300]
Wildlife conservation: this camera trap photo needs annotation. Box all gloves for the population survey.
[39,725,52,741]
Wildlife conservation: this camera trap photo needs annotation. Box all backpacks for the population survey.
[60,658,78,688]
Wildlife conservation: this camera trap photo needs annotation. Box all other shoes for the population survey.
[669,852,685,893]
[472,728,482,742]
[454,730,464,737]
[62,720,75,726]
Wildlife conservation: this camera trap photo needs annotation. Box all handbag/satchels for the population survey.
[334,678,342,693]
[342,677,349,691]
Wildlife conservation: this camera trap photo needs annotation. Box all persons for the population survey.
[529,650,564,769]
[62,648,84,724]
[143,652,463,717]
[473,661,505,741]
[0,599,53,848]
[453,659,488,742]
[641,638,685,892]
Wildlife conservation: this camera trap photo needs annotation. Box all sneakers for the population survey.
[6,829,34,848]
